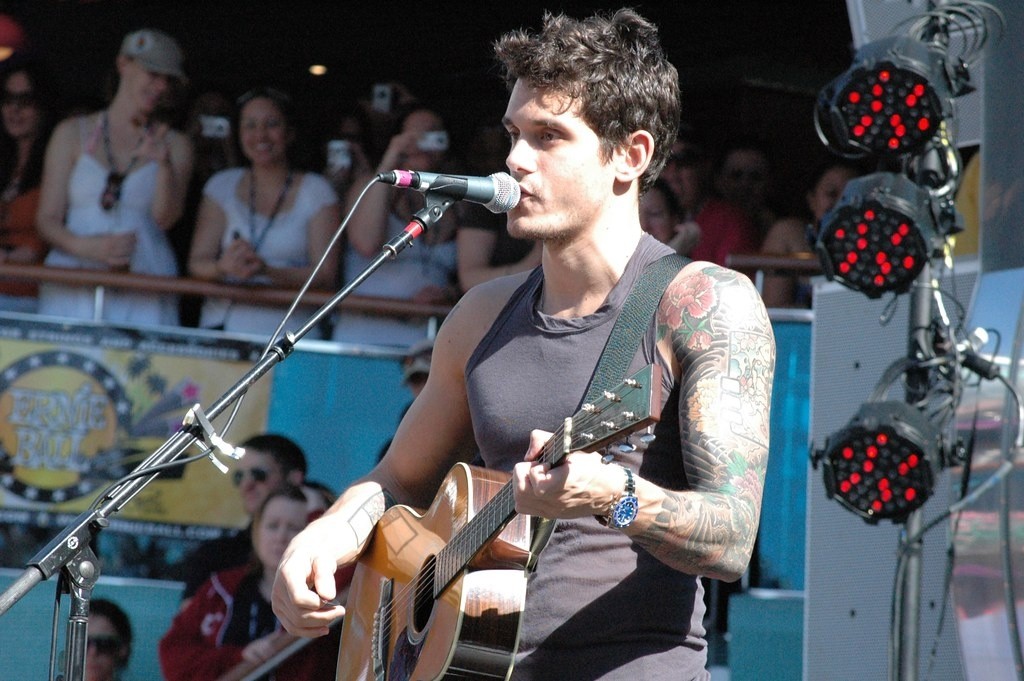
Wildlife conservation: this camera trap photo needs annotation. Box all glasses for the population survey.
[231,464,279,485]
[86,632,126,656]
[0,87,38,108]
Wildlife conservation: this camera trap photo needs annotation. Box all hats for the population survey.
[120,30,188,87]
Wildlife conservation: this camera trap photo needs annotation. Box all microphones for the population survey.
[378,169,521,212]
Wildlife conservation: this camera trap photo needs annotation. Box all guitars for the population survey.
[334,361,664,680]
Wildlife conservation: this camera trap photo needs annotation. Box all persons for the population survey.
[1,28,877,342]
[180,434,307,600]
[270,8,776,681]
[300,481,338,524]
[84,599,131,681]
[158,485,335,681]
[376,340,434,464]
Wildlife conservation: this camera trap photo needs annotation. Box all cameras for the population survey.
[414,131,450,151]
[327,141,352,170]
[371,84,393,114]
[195,114,229,140]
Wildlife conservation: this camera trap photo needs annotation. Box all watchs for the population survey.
[595,468,639,530]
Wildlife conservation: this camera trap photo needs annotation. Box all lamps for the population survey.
[821,399,945,529]
[817,173,934,299]
[812,34,953,161]
